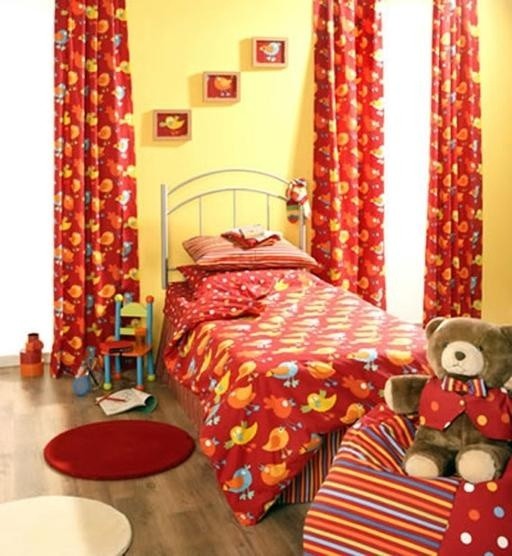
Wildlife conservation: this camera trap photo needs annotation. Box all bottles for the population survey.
[87,346,104,389]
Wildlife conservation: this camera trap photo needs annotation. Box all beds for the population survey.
[160,167,438,534]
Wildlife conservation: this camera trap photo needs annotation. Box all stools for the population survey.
[295,404,511,556]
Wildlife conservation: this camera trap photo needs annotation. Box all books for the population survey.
[96,387,158,416]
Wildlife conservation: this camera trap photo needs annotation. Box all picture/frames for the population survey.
[152,108,192,142]
[252,37,289,68]
[202,69,241,103]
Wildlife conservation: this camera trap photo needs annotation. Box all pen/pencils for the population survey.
[105,397,125,401]
[95,391,113,405]
[78,361,100,387]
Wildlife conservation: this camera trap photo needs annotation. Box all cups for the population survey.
[73,375,90,397]
[136,328,147,350]
[27,333,38,344]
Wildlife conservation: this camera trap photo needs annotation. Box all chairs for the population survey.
[98,292,155,392]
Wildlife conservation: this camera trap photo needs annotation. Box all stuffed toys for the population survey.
[384,317,512,483]
[72,347,99,396]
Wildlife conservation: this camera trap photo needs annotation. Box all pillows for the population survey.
[180,231,320,273]
[174,264,297,301]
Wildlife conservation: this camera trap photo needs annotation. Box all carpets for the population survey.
[42,418,196,483]
[0,493,136,556]
